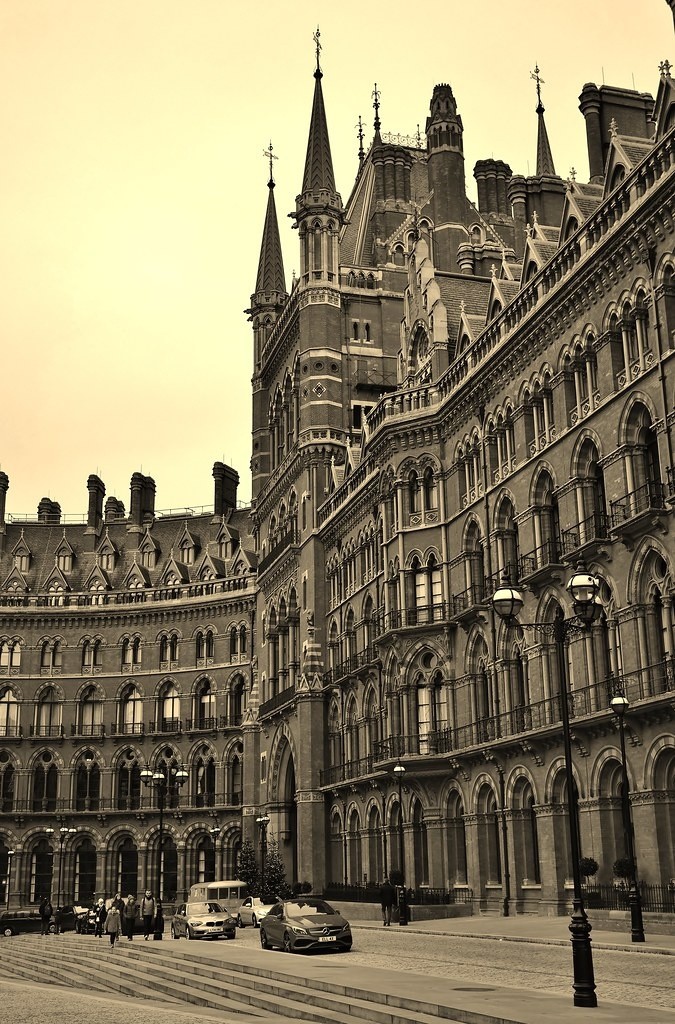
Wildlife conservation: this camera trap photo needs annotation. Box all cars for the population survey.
[1,912,59,937]
[54,901,93,933]
[74,909,111,937]
[261,895,353,953]
[238,896,283,930]
[172,902,234,940]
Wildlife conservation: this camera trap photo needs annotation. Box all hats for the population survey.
[128,894,134,900]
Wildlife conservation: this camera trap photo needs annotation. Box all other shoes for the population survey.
[111,944,114,948]
[128,938,132,941]
[41,933,50,935]
[115,936,119,941]
[383,921,391,926]
[144,934,149,941]
[94,932,102,938]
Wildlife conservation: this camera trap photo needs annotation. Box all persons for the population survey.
[104,905,121,948]
[39,896,54,936]
[140,888,157,941]
[123,894,138,941]
[381,878,393,926]
[110,893,124,941]
[93,898,106,939]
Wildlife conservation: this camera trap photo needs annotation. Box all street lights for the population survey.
[608,686,646,945]
[255,808,272,900]
[44,814,77,914]
[489,574,609,1010]
[7,848,16,912]
[138,767,192,937]
[389,755,416,926]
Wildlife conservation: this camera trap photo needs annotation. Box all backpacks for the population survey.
[44,903,52,917]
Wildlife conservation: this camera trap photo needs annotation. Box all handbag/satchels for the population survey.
[96,916,100,923]
